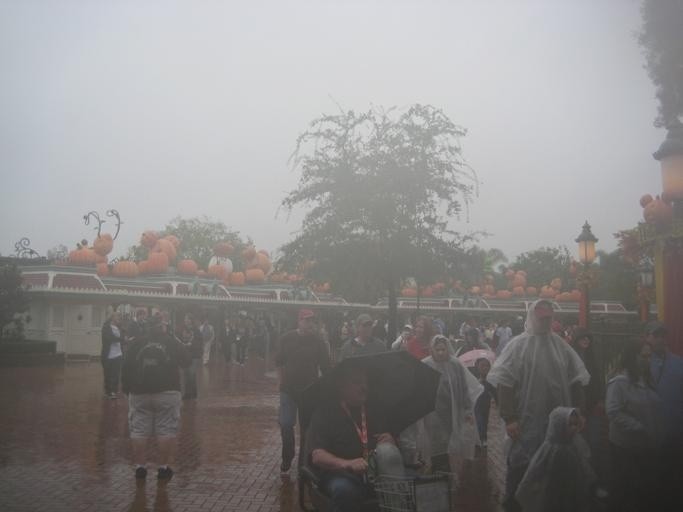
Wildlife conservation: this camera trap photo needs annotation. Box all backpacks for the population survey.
[133,340,174,387]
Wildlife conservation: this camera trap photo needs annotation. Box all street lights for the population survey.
[571,218,600,327]
[636,256,657,322]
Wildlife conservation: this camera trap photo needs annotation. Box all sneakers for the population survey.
[226,354,249,369]
[135,467,147,478]
[104,389,121,401]
[157,466,174,481]
[474,438,488,449]
[281,460,291,471]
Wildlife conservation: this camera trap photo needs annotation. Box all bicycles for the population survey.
[298,456,456,512]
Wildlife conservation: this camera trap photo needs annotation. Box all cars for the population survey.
[568,314,645,350]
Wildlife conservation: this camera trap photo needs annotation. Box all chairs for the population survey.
[292,405,383,511]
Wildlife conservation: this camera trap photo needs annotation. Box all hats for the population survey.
[358,314,372,324]
[299,308,313,320]
[646,321,668,334]
